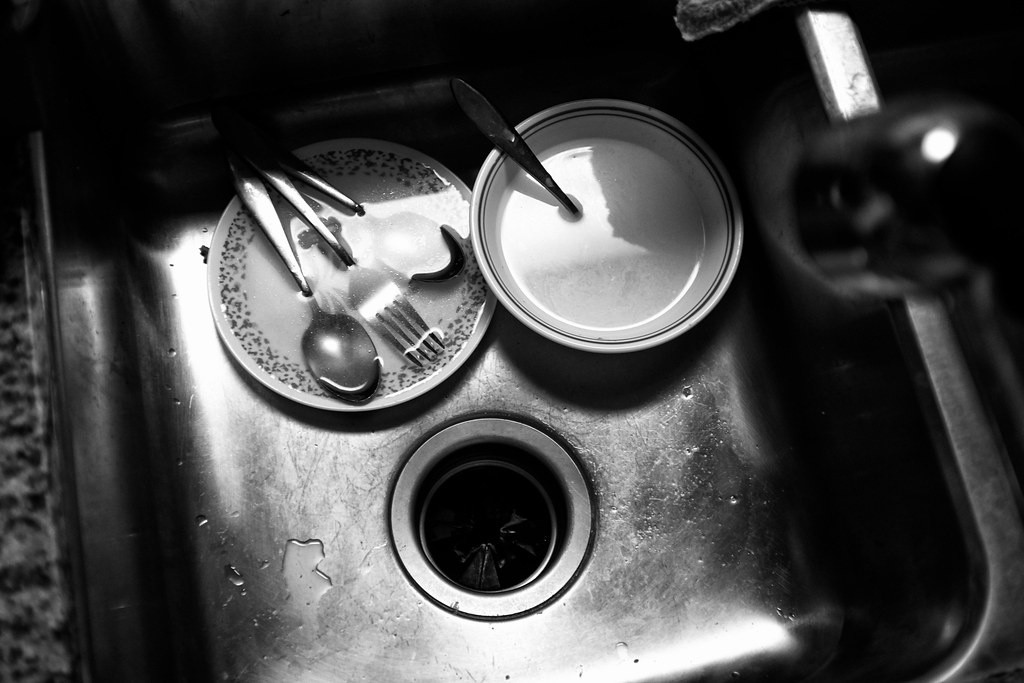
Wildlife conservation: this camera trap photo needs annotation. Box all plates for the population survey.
[207,139,498,412]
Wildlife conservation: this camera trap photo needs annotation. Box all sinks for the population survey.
[0,0,1024,683]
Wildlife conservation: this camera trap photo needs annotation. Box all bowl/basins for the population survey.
[472,99,745,353]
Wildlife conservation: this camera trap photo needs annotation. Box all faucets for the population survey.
[761,81,1024,293]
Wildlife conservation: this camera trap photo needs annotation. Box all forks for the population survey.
[216,122,445,367]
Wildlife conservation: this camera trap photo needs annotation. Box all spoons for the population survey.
[214,138,380,401]
[210,106,466,283]
